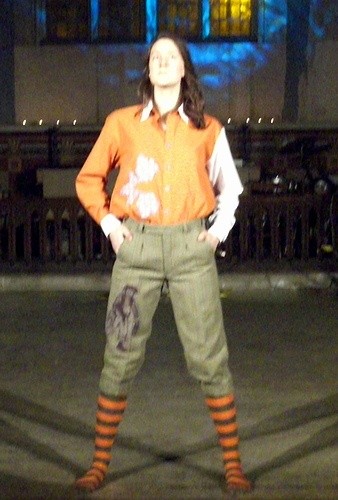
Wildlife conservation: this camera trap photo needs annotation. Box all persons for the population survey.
[72,32,253,492]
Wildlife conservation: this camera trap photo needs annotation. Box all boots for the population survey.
[204,395,251,492]
[76,393,126,491]
